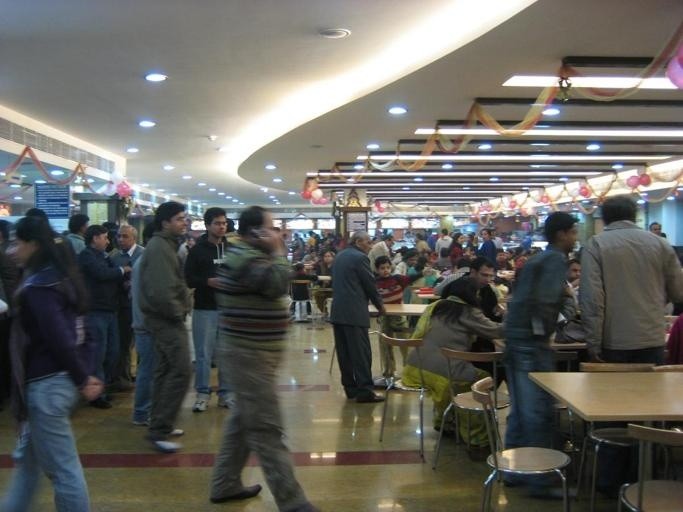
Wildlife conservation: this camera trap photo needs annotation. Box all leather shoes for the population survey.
[346,390,386,403]
[209,484,262,504]
[88,392,115,410]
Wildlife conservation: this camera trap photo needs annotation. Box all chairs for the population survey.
[378,332,458,458]
[432,347,512,470]
[470,377,573,512]
[288,279,313,322]
[652,363,683,479]
[575,360,658,512]
[617,424,682,511]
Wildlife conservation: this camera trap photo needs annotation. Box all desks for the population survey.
[368,303,431,316]
[493,338,590,386]
[415,290,442,300]
[527,372,683,482]
[318,276,333,317]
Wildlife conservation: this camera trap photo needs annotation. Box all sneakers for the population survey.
[595,483,618,499]
[469,445,499,463]
[168,428,185,437]
[146,439,182,454]
[528,484,565,499]
[132,417,152,427]
[503,478,528,487]
[216,396,238,410]
[434,423,457,437]
[191,399,211,412]
